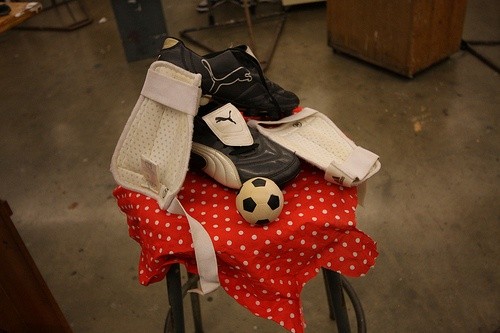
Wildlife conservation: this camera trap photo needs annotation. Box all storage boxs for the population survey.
[325,0,468,77]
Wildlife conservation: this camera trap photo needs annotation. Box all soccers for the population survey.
[235,177,285,225]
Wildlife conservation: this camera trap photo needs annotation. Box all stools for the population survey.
[119,162,367,333]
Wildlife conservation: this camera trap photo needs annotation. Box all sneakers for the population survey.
[157,38,299,114]
[187,101,301,189]
